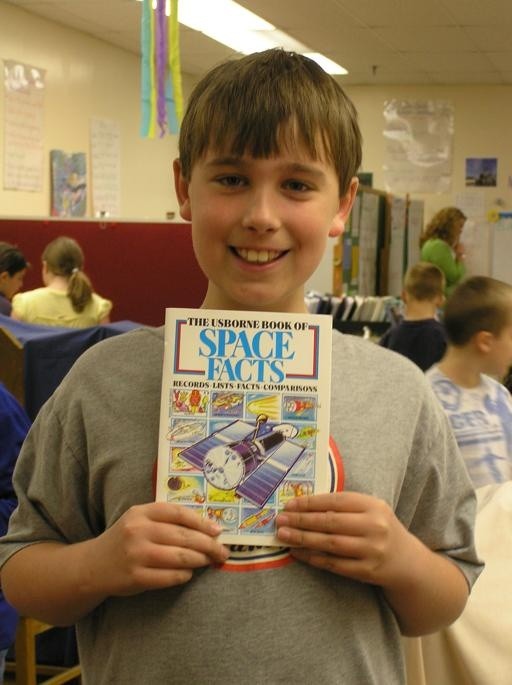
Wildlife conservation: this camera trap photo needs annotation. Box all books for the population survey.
[154,305,333,548]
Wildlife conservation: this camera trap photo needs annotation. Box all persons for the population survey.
[0,242,31,319]
[0,47,486,684]
[10,236,112,330]
[420,206,468,322]
[424,275,511,492]
[1,382,33,684]
[378,261,448,374]
